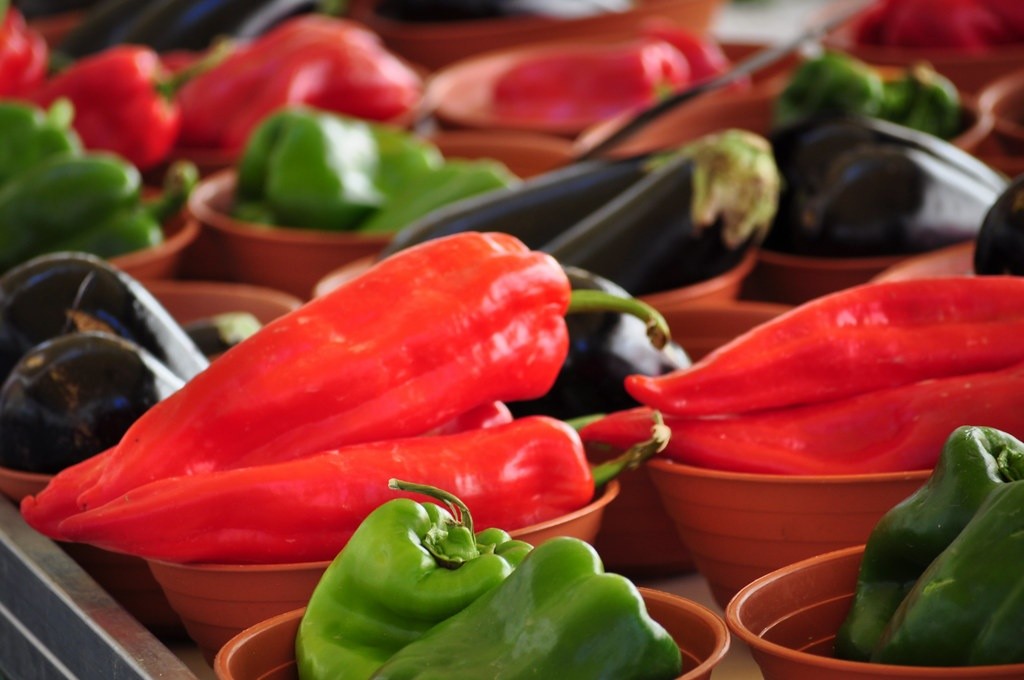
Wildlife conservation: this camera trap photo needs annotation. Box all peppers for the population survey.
[20,231,1021,680]
[0,0,1019,260]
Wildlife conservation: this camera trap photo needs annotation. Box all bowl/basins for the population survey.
[0,281,310,651]
[214,588,731,679]
[644,455,932,611]
[820,1,1022,89]
[313,248,759,315]
[862,233,975,287]
[136,461,621,671]
[756,245,898,304]
[572,72,1001,165]
[584,304,799,581]
[343,0,721,59]
[725,543,1024,680]
[103,177,210,283]
[425,31,812,140]
[978,68,1020,158]
[377,60,433,135]
[182,129,576,294]
